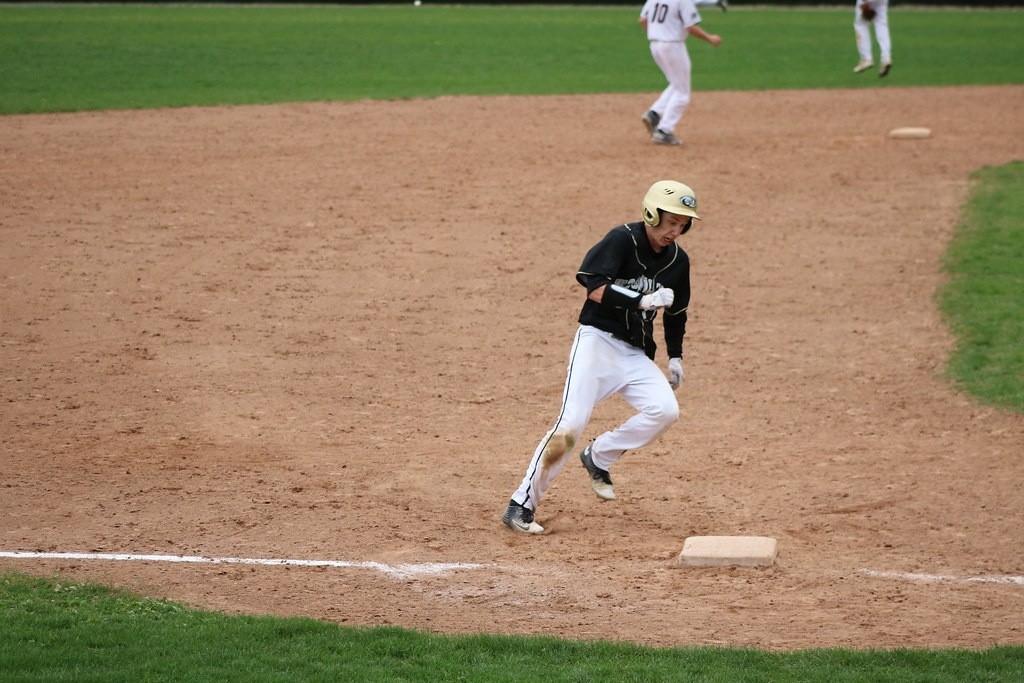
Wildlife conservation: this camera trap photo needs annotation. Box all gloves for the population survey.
[668,358,685,391]
[640,287,675,310]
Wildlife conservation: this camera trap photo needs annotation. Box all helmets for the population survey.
[641,180,701,235]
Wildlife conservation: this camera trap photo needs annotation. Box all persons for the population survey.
[502,180,703,533]
[639,0,722,145]
[853,0,893,77]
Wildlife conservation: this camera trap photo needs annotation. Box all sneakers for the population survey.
[853,61,873,72]
[641,111,660,136]
[502,506,544,533]
[878,60,891,78]
[651,131,682,145]
[579,443,616,500]
